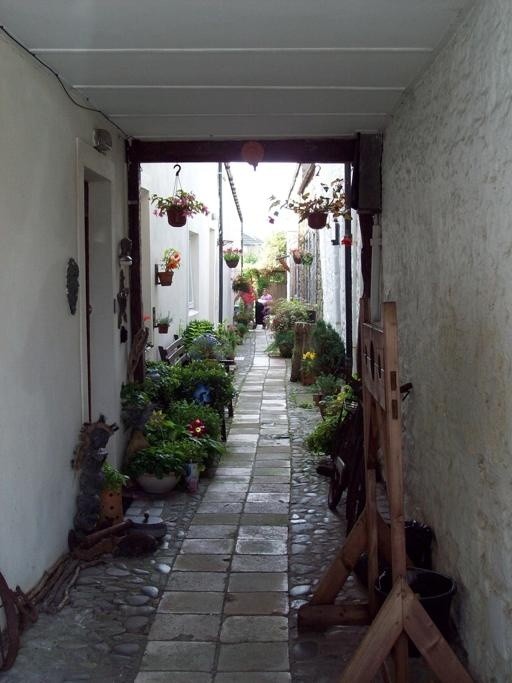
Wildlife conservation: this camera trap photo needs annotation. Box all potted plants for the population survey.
[263,294,350,424]
[224,251,241,269]
[99,462,130,523]
[266,177,355,230]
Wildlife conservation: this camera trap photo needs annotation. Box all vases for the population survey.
[165,207,189,227]
[159,269,175,286]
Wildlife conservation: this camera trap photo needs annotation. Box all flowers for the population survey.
[118,316,248,496]
[161,247,182,272]
[149,190,210,218]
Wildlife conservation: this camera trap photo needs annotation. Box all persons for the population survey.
[260,288,273,329]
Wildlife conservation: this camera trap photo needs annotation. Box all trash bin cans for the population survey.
[387,522,432,569]
[255,303,265,325]
[375,566,458,657]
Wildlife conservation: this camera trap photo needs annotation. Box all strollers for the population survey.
[253,297,270,330]
